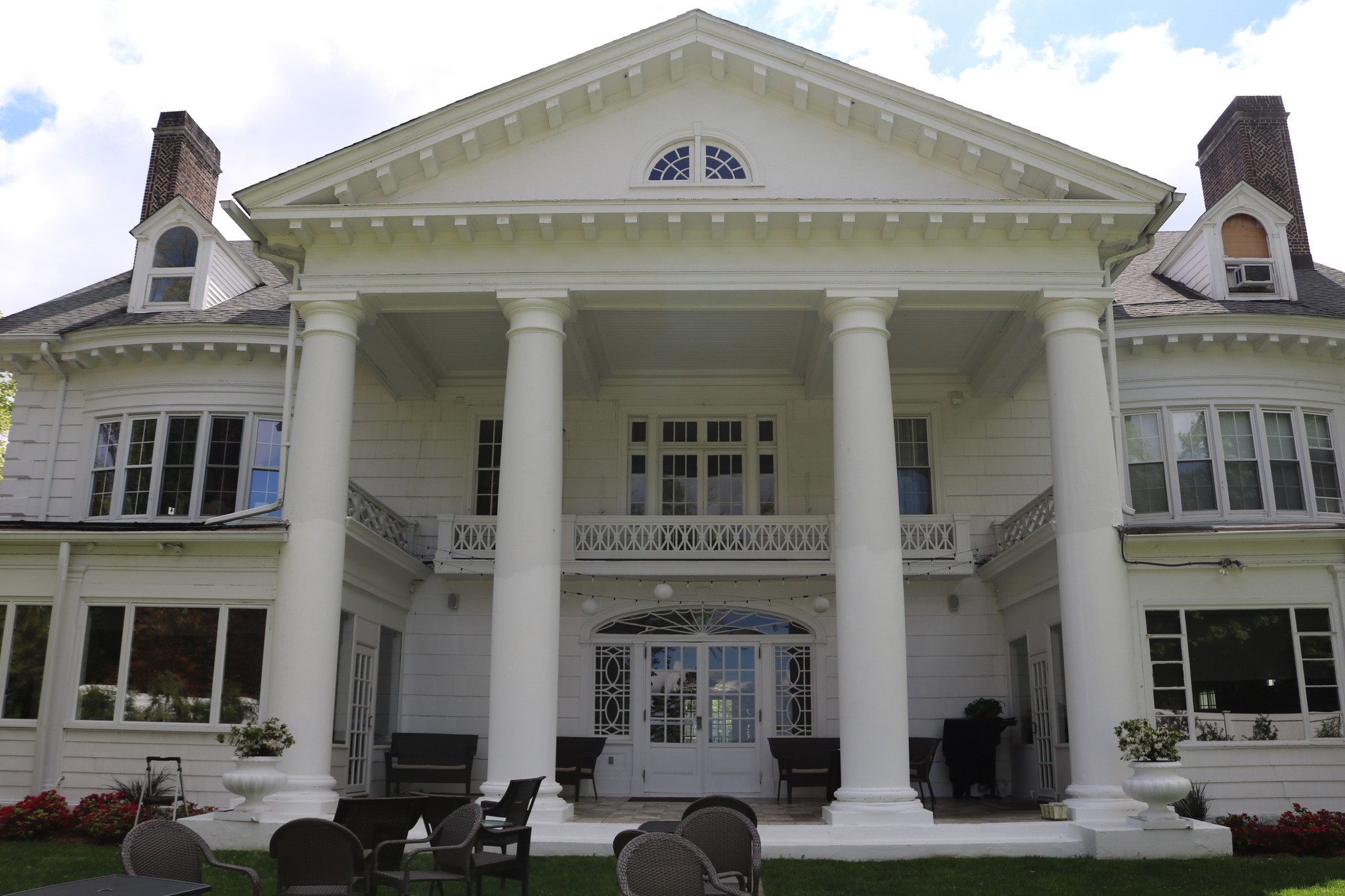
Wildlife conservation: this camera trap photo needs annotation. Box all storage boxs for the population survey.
[1038,803,1069,822]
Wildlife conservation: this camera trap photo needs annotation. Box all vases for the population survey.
[222,756,286,811]
[1121,762,1192,821]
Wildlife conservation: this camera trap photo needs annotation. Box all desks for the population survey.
[637,818,681,834]
[3,874,212,896]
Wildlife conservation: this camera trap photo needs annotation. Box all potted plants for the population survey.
[965,698,1006,721]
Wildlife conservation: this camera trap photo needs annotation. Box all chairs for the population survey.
[908,735,940,807]
[122,823,261,896]
[614,795,766,896]
[270,775,546,896]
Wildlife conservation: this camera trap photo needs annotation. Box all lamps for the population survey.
[1219,559,1248,575]
[654,583,673,602]
[158,542,182,555]
[811,595,831,614]
[581,595,598,617]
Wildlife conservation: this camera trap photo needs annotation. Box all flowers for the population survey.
[0,791,218,843]
[1115,716,1185,763]
[217,718,295,756]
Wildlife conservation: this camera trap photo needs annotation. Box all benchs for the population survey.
[556,736,608,801]
[384,732,479,795]
[767,737,840,804]
